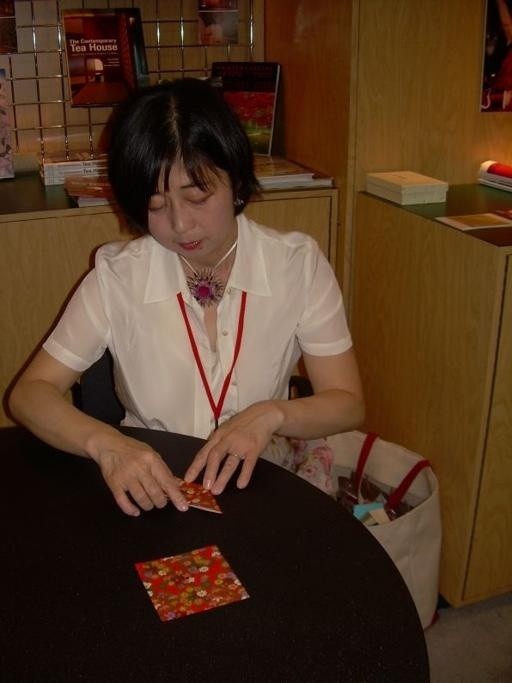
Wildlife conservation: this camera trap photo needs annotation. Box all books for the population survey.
[210,59,282,157]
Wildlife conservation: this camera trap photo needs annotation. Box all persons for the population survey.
[2,77,369,521]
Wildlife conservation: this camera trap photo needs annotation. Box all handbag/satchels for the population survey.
[305,430,443,630]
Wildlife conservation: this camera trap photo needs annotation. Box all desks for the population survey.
[0,425,430,683]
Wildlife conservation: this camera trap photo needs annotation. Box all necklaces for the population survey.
[179,238,238,308]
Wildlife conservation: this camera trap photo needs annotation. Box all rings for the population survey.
[230,452,242,461]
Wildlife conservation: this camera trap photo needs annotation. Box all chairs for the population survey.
[77,347,124,424]
